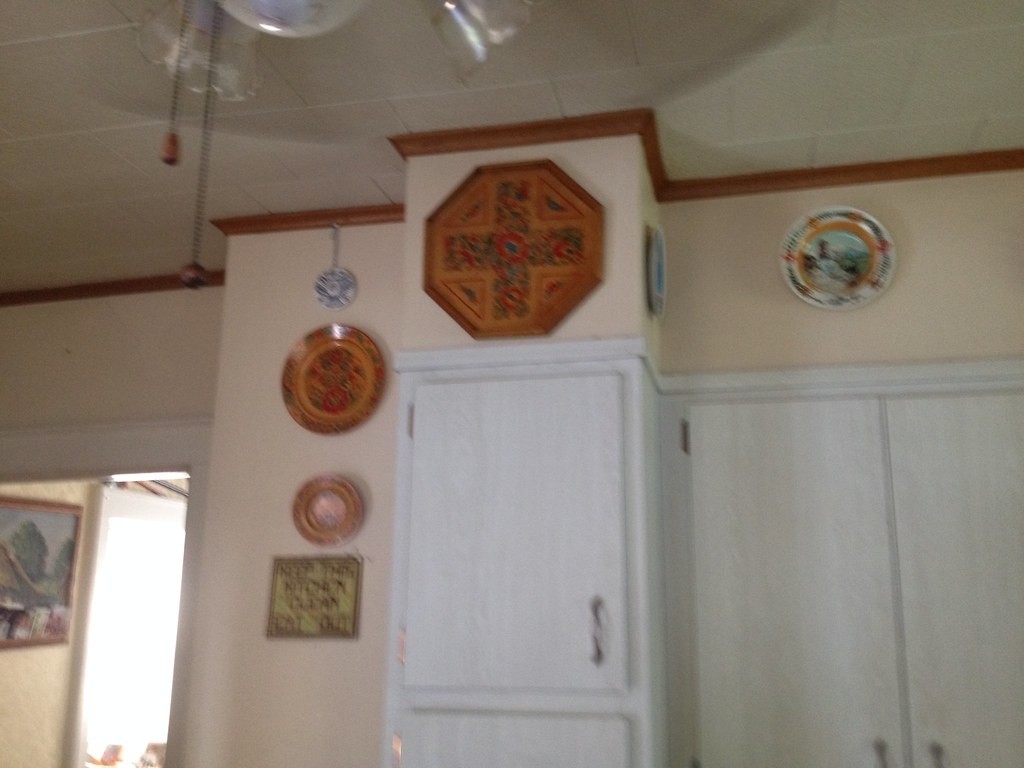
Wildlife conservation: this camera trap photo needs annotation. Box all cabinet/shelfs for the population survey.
[383,336,1024,768]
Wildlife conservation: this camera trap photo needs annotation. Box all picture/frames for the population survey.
[0,495,85,650]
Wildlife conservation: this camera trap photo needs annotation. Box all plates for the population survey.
[282,325,385,435]
[292,475,365,547]
[780,206,895,310]
[647,230,665,314]
